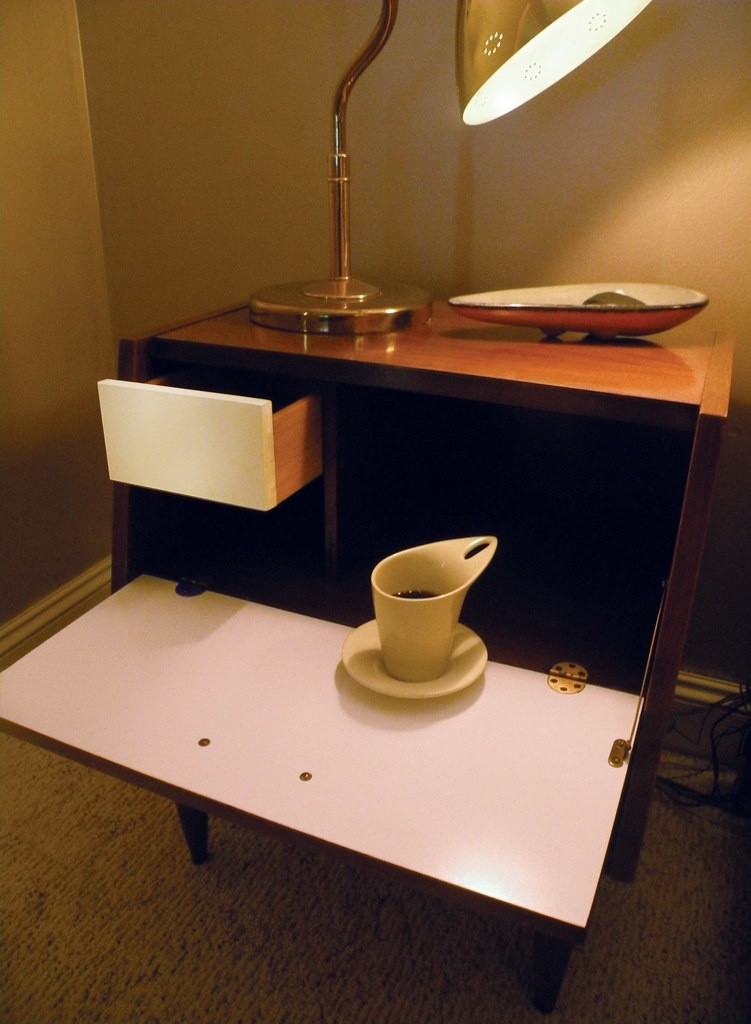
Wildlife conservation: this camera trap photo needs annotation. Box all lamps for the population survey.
[248,0,655,334]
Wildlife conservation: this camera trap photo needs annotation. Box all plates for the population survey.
[342,617,487,699]
[449,282,709,338]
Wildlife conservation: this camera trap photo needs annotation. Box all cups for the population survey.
[371,538,498,681]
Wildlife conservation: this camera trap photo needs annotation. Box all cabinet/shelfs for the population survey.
[0,299,734,1015]
